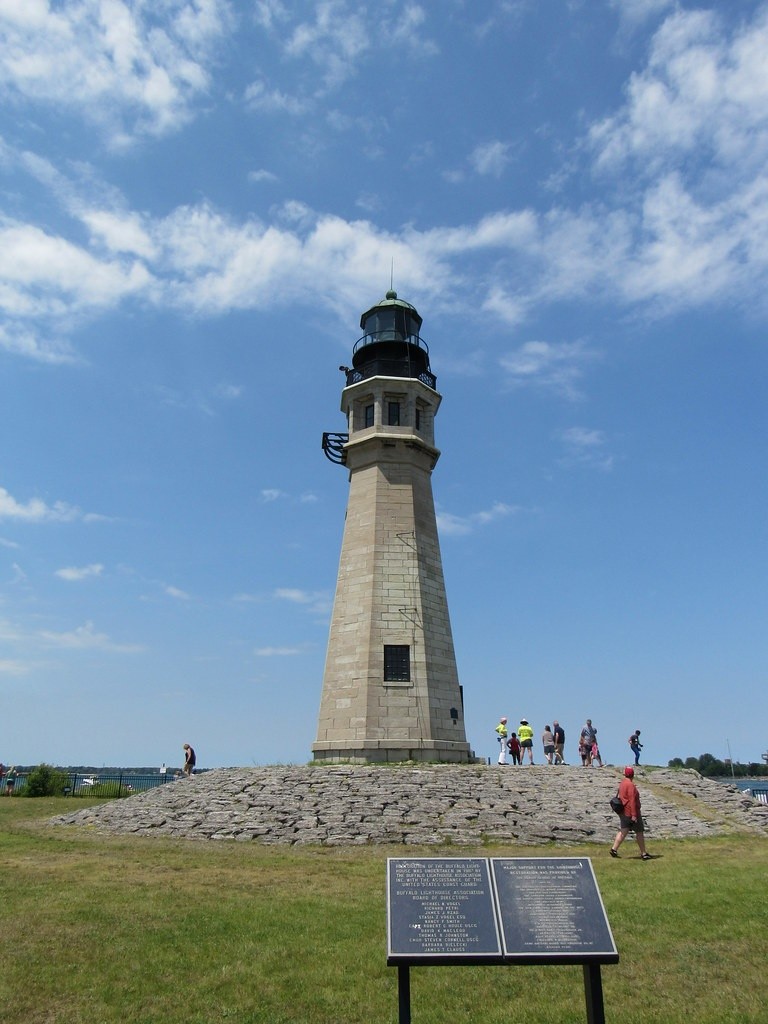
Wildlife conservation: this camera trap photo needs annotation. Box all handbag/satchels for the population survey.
[610,797,625,814]
[497,737,501,742]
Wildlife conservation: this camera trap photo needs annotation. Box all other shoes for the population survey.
[641,853,654,859]
[609,849,621,858]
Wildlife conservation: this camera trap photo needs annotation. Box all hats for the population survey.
[519,719,529,724]
[499,718,507,723]
[625,767,634,776]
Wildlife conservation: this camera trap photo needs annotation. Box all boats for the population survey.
[124,784,135,791]
[83,775,100,786]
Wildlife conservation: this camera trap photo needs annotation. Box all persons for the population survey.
[609,767,652,859]
[553,720,566,765]
[495,718,509,765]
[4,766,19,798]
[173,768,197,781]
[579,719,604,767]
[507,733,522,765]
[628,730,643,766]
[517,718,535,765]
[0,763,6,788]
[542,725,555,764]
[183,743,196,777]
